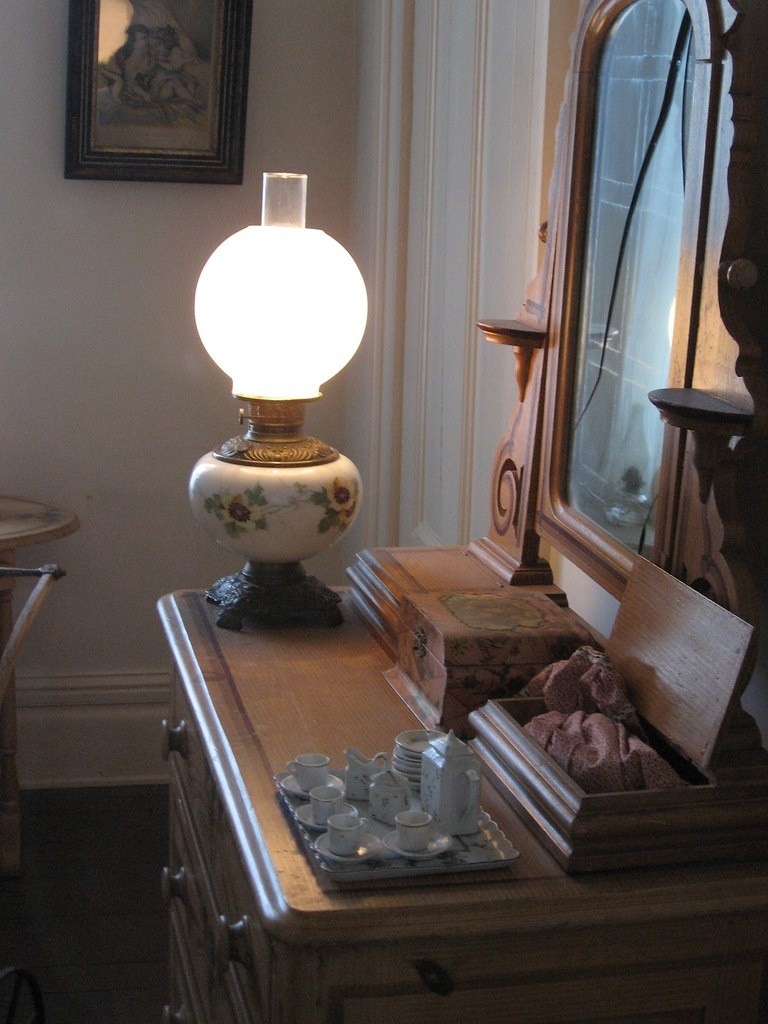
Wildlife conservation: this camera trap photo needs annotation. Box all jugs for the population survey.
[341,745,389,801]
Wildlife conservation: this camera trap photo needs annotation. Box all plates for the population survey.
[280,773,345,800]
[387,729,448,790]
[294,804,358,832]
[314,832,384,864]
[382,830,453,861]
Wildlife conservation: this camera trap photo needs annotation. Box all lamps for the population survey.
[188,172,368,633]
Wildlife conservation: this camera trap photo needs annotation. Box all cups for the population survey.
[285,753,330,792]
[309,785,345,826]
[394,809,433,852]
[327,814,366,857]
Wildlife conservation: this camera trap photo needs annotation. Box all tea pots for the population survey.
[418,729,481,836]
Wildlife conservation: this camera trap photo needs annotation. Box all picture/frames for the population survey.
[63,0,254,185]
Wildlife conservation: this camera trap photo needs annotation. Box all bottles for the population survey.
[366,770,412,824]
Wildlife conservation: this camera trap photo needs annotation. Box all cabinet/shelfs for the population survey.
[155,585,768,1024]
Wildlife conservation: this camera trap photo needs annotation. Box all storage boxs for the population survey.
[381,589,604,741]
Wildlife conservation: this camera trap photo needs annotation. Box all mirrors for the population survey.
[534,0,725,603]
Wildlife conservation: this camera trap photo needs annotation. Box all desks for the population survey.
[0,495,81,880]
